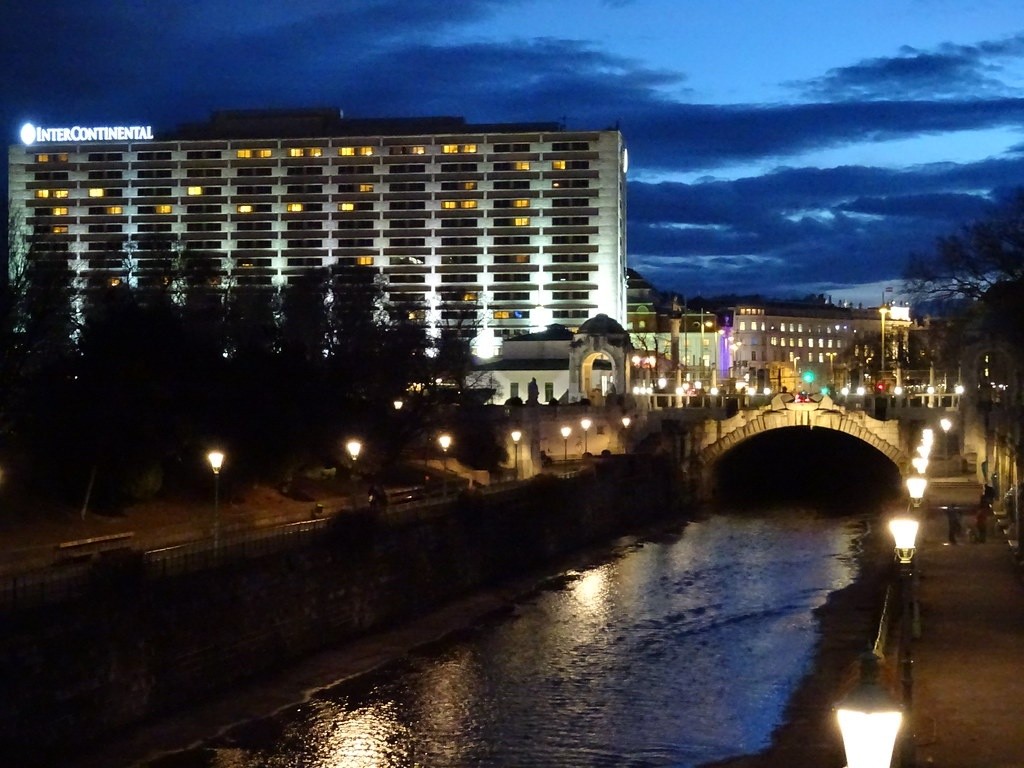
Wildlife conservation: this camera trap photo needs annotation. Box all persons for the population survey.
[528,378,538,404]
[946,501,959,543]
[541,451,549,469]
[984,482,995,510]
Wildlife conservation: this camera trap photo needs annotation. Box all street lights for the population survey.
[939,418,952,478]
[700,308,714,394]
[711,317,725,394]
[580,419,592,453]
[207,448,227,558]
[905,475,930,642]
[889,518,923,768]
[346,438,364,509]
[560,426,571,479]
[878,307,889,369]
[620,415,632,454]
[438,435,452,501]
[510,430,523,483]
